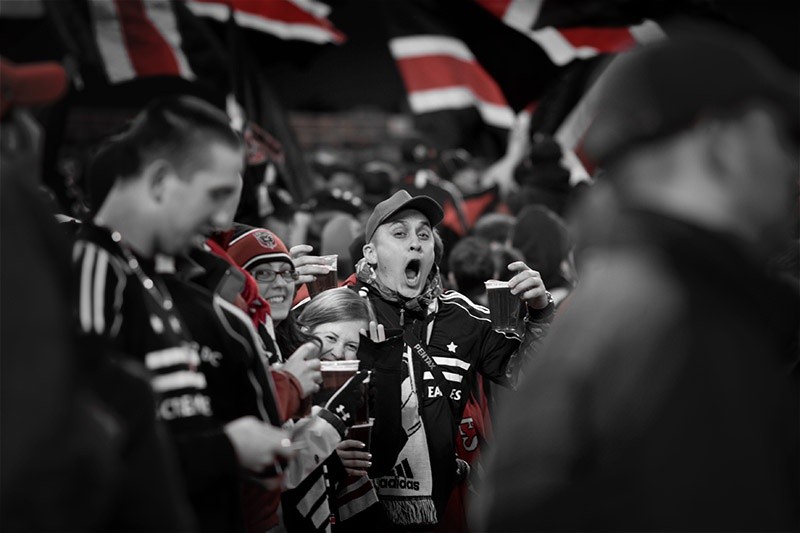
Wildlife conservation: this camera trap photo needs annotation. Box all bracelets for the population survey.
[525,290,555,319]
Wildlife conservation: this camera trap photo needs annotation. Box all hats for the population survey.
[365,189,444,243]
[226,222,296,272]
[0,61,65,117]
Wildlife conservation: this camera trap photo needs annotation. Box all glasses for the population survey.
[252,270,300,282]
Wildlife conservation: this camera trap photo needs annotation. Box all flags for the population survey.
[388,34,516,130]
[476,0,541,34]
[89,0,196,84]
[185,0,346,45]
[525,19,667,67]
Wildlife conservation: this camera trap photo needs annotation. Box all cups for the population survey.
[306,254,338,300]
[345,423,373,470]
[320,360,361,394]
[484,281,521,331]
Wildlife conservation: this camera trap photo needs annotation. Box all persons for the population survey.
[0,32,800,533]
